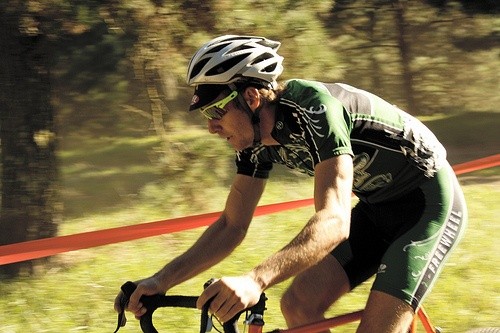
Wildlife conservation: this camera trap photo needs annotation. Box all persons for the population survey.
[114,33,470,333]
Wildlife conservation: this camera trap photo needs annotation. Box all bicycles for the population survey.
[114,282,500,333]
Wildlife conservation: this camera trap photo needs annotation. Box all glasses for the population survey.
[199,86,241,122]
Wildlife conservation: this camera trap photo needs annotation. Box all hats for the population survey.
[189,84,228,110]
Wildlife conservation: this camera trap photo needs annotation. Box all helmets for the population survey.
[186,31,285,86]
